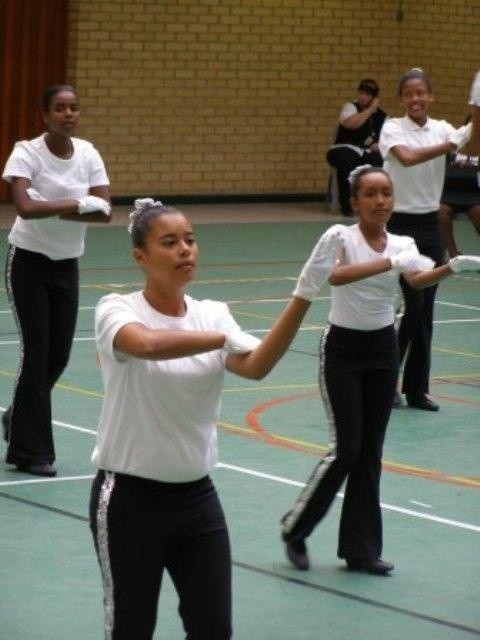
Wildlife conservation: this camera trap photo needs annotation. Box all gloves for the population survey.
[291,228,345,304]
[75,195,112,217]
[447,255,480,273]
[26,187,49,203]
[468,71,479,106]
[383,249,436,275]
[447,121,474,154]
[221,328,262,356]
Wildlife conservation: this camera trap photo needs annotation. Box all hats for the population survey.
[357,79,380,99]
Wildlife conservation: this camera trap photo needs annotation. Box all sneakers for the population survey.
[391,390,439,411]
[345,556,395,573]
[281,510,310,571]
[1,405,57,476]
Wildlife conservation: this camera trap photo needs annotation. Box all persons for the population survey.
[440,112,480,274]
[281,162,480,576]
[325,77,391,216]
[89,196,343,639]
[1,82,112,477]
[377,68,480,410]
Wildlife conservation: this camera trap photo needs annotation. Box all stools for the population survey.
[325,169,339,215]
